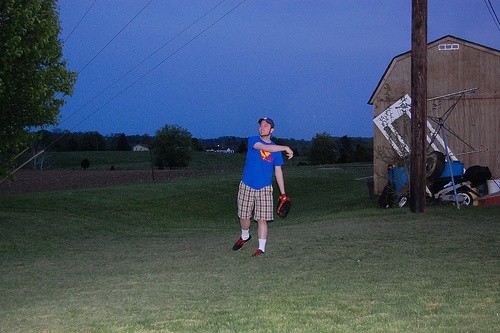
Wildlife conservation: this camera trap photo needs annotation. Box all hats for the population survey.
[258,117,274,128]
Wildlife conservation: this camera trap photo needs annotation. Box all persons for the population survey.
[232,117,293,257]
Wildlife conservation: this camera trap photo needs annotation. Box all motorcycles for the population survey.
[397,163,481,206]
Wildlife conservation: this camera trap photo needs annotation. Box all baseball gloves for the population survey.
[277,193,291,218]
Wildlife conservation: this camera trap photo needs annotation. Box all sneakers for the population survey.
[233,234,252,252]
[251,248,266,258]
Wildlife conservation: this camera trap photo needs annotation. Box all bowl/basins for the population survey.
[479,191,500,208]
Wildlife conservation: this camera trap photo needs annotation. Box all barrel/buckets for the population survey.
[388,166,408,200]
[487,180,500,194]
[439,160,464,177]
[468,188,479,206]
